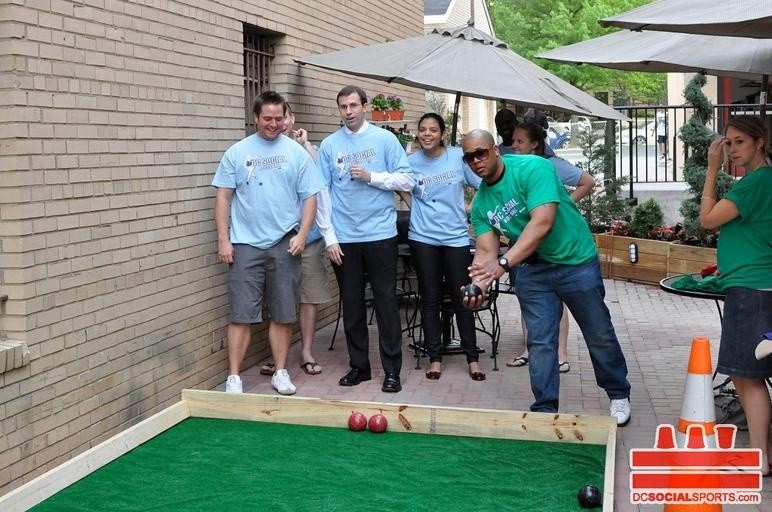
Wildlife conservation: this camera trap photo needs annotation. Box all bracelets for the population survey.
[700,197,715,200]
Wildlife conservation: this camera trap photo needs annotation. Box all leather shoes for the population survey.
[339,371,369,386]
[382,375,401,392]
[467,365,486,381]
[426,370,440,380]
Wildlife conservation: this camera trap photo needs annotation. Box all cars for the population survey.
[546,115,657,150]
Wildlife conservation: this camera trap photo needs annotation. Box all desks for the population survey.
[658,273,772,423]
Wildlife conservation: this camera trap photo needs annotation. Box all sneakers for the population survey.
[225,374,242,393]
[610,398,630,427]
[270,368,296,395]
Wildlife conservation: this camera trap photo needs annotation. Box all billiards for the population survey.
[579,484,600,508]
[369,414,388,432]
[348,412,366,430]
[462,283,482,306]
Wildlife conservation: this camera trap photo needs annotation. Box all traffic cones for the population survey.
[662,336,726,510]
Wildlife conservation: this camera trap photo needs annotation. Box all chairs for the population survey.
[329,210,514,373]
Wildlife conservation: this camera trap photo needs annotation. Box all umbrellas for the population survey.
[533,28,772,117]
[597,0,772,39]
[292,17,633,146]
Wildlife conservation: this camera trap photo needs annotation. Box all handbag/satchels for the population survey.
[715,387,748,431]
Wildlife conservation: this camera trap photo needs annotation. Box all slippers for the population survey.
[301,362,322,375]
[260,364,276,375]
[559,362,569,372]
[506,355,529,367]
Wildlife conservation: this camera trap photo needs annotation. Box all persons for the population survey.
[700,114,772,478]
[207,83,633,423]
[651,104,668,159]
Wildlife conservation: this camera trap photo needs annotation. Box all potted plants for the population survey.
[371,94,389,120]
[387,95,405,120]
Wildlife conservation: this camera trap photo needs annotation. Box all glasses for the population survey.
[463,147,494,165]
